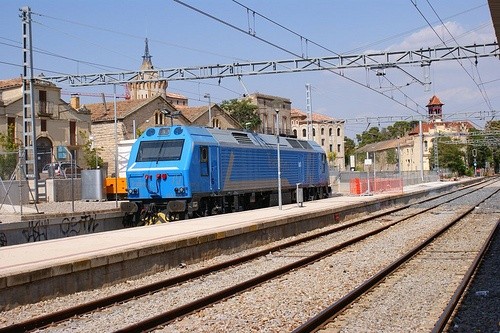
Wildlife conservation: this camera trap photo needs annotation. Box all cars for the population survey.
[41,162,80,177]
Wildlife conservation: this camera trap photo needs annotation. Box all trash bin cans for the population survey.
[295,182,305,208]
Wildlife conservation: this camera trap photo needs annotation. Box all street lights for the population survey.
[160,108,182,125]
[204,92,213,127]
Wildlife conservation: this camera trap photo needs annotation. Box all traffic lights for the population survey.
[472,149,477,158]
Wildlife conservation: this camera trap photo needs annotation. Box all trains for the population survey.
[120,125,332,226]
